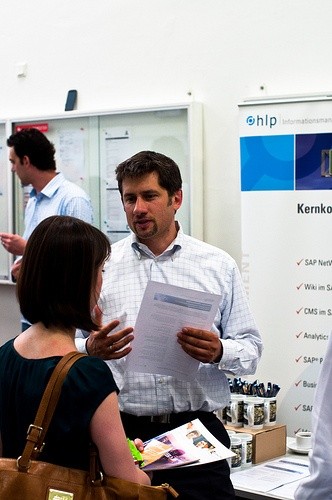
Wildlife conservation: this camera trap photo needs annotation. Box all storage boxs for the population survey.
[224,424,286,464]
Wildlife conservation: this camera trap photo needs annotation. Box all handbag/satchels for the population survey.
[0,352,179,500]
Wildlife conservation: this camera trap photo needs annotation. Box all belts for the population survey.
[122,410,198,423]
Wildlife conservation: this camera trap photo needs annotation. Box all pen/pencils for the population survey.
[227,378,280,398]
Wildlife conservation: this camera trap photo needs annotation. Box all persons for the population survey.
[75,151,265,500]
[0,216,152,486]
[187,430,206,449]
[0,128,94,331]
[294,332,332,500]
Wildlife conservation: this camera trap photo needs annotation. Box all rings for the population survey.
[110,345,115,352]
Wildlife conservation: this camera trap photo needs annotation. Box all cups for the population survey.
[226,430,253,471]
[218,396,279,429]
[296,431,313,448]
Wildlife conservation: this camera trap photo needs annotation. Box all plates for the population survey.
[287,443,310,454]
